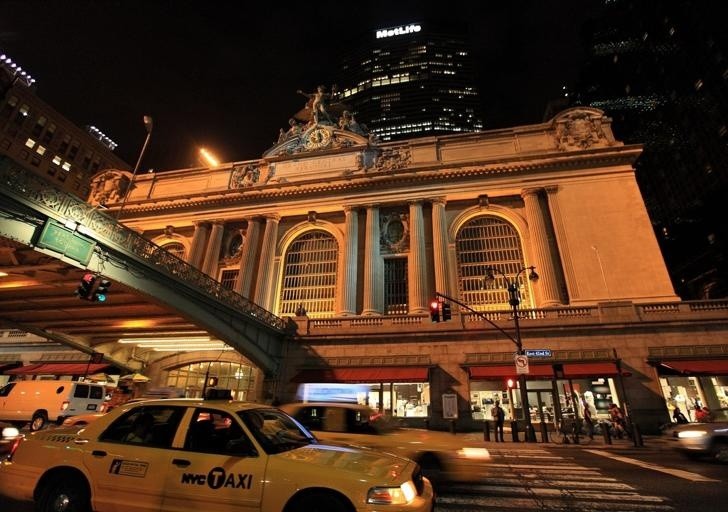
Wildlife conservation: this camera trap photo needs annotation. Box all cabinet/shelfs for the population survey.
[369,391,397,416]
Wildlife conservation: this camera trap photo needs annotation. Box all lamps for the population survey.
[295,303,306,316]
[164,226,175,238]
[308,211,318,222]
[206,377,218,387]
[479,194,489,209]
[235,364,244,380]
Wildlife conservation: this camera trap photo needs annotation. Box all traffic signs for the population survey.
[526,349,552,357]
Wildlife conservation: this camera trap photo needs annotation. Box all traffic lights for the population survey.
[73,272,111,303]
[208,377,218,387]
[430,299,451,323]
[505,376,517,389]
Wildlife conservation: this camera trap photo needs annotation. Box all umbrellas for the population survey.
[85,373,116,384]
[118,372,153,385]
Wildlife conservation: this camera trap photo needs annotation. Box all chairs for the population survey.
[530,403,575,423]
[116,411,248,458]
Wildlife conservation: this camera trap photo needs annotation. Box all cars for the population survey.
[667,408,728,463]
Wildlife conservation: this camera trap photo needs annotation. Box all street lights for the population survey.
[482,266,539,441]
[114,115,154,222]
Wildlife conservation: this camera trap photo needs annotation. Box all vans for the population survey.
[0,380,106,431]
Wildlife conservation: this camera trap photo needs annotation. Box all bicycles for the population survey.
[550,420,591,445]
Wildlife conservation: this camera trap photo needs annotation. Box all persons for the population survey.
[702,407,714,423]
[119,413,158,445]
[119,381,133,394]
[607,403,624,441]
[672,408,688,425]
[295,83,336,126]
[583,403,594,440]
[338,108,366,137]
[693,405,704,423]
[284,118,302,139]
[490,400,504,443]
[237,416,280,455]
[608,404,633,441]
